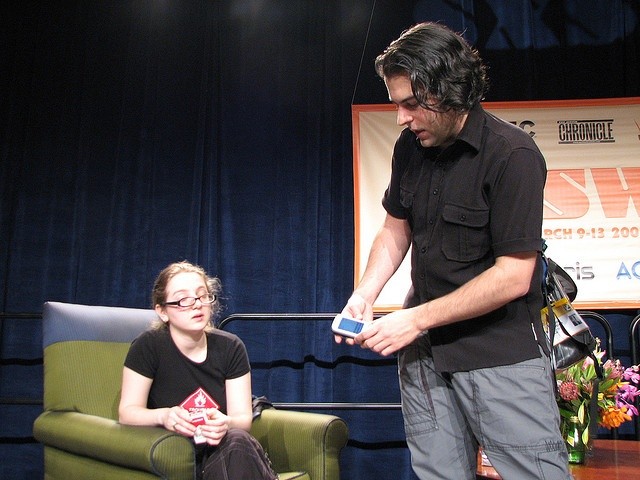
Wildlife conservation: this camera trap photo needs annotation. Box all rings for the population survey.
[173,422,178,430]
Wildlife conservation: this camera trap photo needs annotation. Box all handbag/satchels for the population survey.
[543,257,604,383]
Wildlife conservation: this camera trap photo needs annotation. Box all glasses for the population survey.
[161,293,218,307]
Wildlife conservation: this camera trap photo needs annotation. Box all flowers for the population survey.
[551,334,640,464]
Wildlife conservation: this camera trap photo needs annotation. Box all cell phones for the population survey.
[330,313,372,340]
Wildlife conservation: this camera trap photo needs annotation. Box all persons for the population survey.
[118,259,281,480]
[331,20,573,480]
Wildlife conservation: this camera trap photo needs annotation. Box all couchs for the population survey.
[33,301,350,479]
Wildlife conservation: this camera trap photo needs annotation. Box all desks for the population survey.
[476,433,640,479]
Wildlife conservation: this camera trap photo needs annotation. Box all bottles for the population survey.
[565,416,585,464]
